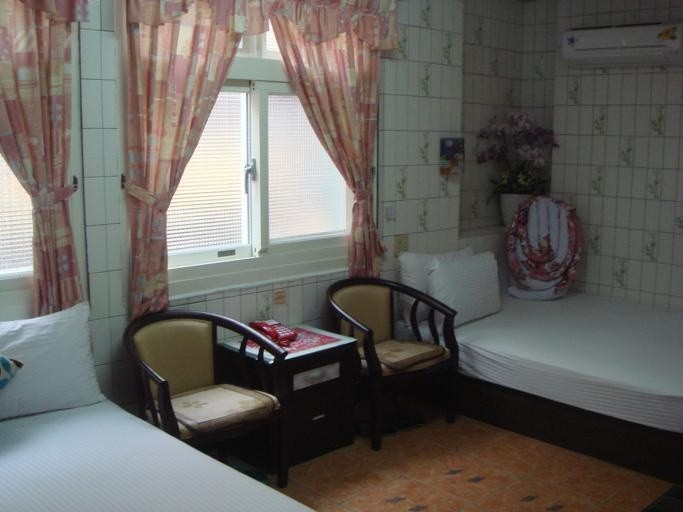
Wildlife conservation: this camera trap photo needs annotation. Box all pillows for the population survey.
[429,252,500,336]
[0,353,23,392]
[0,300,106,420]
[399,246,473,330]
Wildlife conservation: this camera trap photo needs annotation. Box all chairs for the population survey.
[326,272,458,448]
[123,308,289,489]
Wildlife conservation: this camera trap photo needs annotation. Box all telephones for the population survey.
[248,319,298,343]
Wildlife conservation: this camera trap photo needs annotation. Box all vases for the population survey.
[497,188,535,229]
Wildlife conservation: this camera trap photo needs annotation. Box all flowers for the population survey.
[476,110,562,204]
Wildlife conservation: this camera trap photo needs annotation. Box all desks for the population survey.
[213,316,363,470]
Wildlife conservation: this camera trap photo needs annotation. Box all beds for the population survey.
[391,291,681,485]
[2,396,326,512]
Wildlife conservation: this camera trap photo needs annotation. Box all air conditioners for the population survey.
[560,20,682,68]
